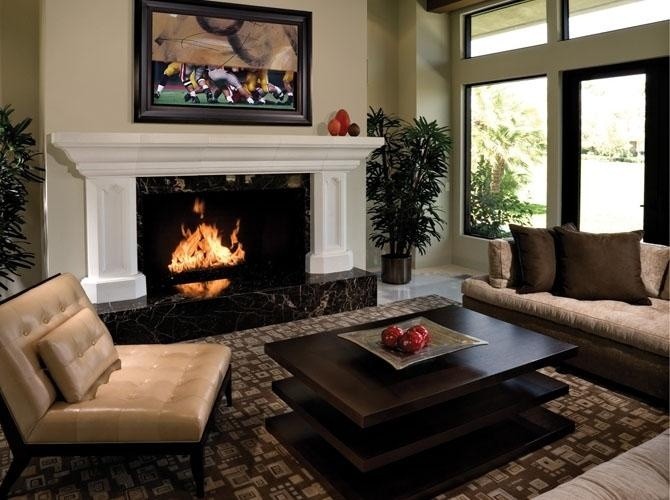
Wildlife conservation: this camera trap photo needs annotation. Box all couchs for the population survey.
[528,421,670,499]
[456,218,670,422]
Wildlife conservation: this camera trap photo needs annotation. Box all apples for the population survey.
[381,323,432,356]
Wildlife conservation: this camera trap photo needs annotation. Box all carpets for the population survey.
[0,290,670,500]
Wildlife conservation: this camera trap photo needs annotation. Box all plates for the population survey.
[337,316,490,374]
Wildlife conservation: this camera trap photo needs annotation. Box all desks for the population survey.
[258,300,585,499]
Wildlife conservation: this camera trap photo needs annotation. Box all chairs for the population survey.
[0,271,240,499]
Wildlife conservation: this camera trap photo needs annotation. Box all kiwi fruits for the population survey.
[348,123,361,136]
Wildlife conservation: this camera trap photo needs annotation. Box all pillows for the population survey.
[549,223,655,308]
[635,237,670,301]
[659,267,670,308]
[505,219,581,296]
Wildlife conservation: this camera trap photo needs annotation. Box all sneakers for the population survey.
[155,86,293,106]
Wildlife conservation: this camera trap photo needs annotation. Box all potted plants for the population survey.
[364,100,453,286]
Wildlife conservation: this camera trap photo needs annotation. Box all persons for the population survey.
[153,62,294,106]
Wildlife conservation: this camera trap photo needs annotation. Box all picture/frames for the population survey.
[130,0,316,128]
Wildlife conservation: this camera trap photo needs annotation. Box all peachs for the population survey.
[328,120,341,135]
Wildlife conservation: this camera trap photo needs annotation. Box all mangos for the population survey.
[335,110,350,136]
